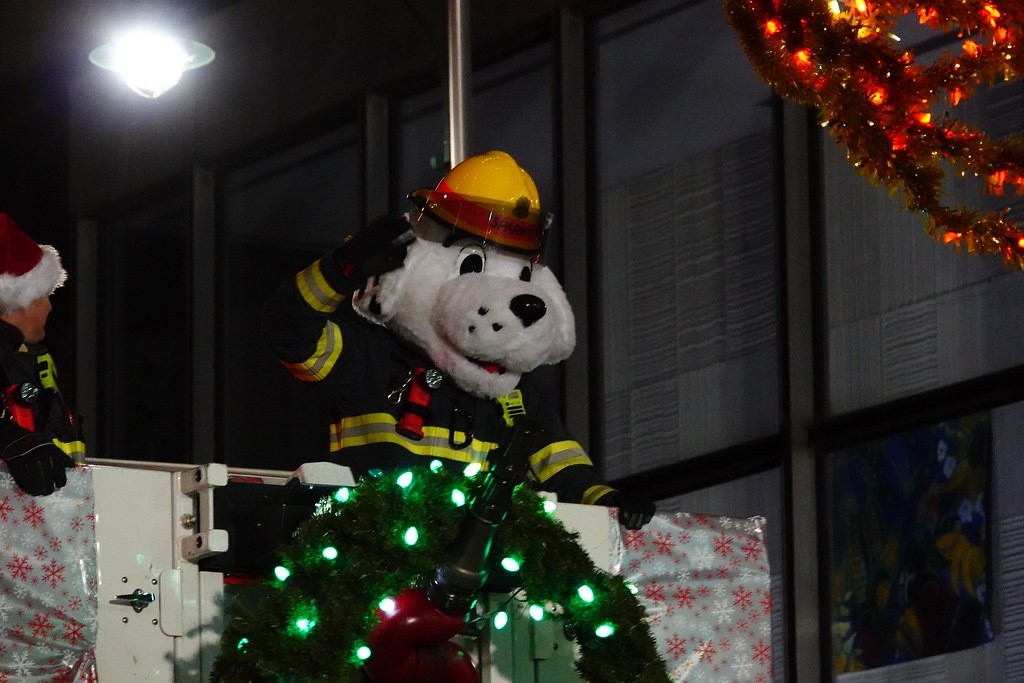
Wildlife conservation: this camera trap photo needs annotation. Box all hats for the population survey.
[0,208,69,310]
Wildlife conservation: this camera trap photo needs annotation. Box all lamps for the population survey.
[89,0,215,100]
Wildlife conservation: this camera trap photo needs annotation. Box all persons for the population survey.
[268,151,657,528]
[0,210,86,496]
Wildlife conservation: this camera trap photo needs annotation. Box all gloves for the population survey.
[0,422,77,496]
